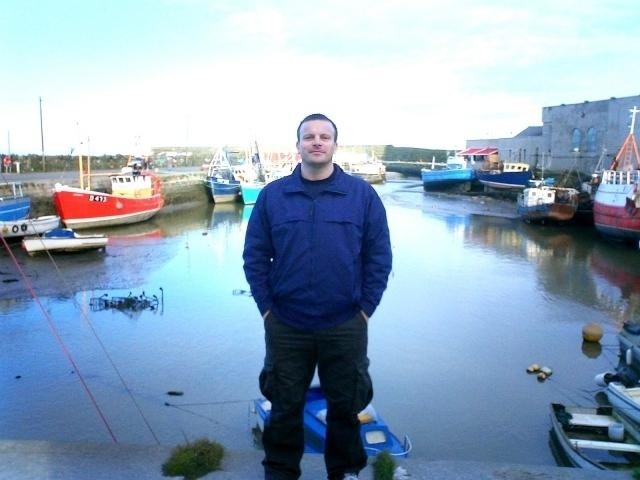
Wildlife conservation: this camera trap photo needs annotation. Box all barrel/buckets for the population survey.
[608,422,625,441]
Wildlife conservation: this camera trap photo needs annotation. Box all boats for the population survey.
[0,214,61,239]
[616,319,640,362]
[205,149,387,205]
[249,383,413,459]
[420,168,477,187]
[478,172,532,190]
[550,406,640,470]
[517,185,580,225]
[22,228,108,256]
[593,366,640,427]
[0,195,31,221]
[53,144,165,229]
[593,106,640,243]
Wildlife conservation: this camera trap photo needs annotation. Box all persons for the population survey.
[242,113,393,480]
